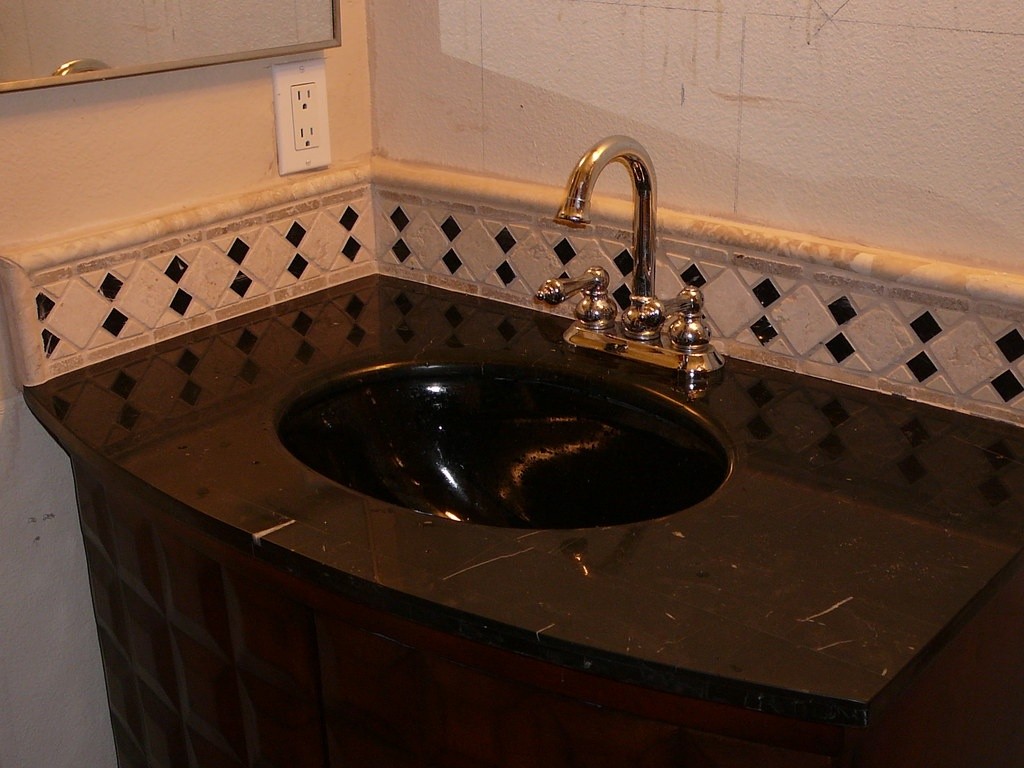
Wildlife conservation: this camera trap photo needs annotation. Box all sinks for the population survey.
[264,343,738,535]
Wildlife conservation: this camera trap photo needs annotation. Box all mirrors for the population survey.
[1,0,343,96]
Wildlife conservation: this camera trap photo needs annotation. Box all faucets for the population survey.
[551,132,666,346]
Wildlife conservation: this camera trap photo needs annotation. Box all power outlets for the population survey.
[269,58,334,177]
